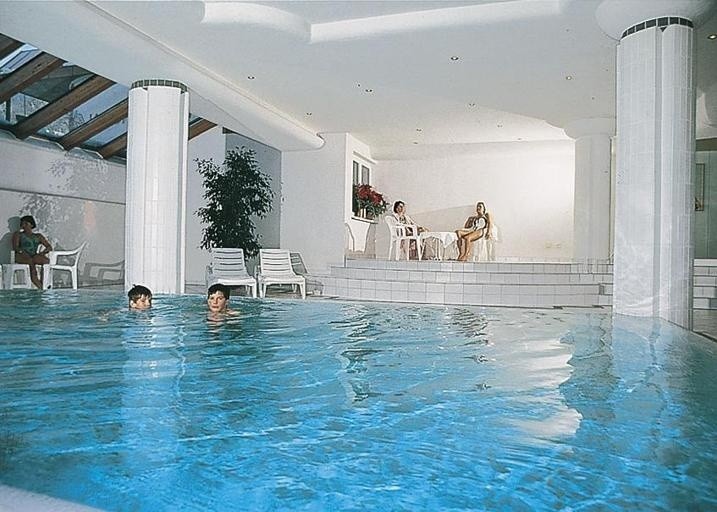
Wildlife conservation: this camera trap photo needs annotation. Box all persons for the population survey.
[12,215,53,293]
[97,284,153,323]
[391,199,430,261]
[454,202,492,262]
[203,283,241,325]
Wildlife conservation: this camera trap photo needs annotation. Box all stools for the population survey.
[2,263,31,290]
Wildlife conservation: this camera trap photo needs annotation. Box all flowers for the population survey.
[353,184,390,215]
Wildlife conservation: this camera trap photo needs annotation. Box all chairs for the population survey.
[385,216,488,261]
[43,240,88,289]
[207,248,324,299]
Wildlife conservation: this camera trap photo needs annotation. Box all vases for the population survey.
[356,207,375,220]
